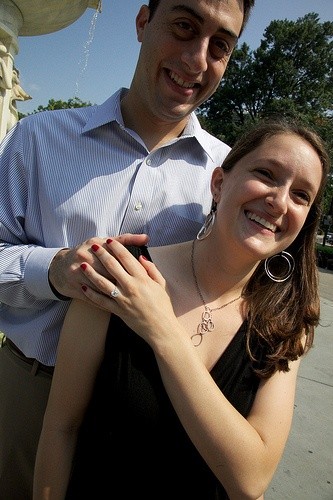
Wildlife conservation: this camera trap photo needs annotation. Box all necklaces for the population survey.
[186,239,252,349]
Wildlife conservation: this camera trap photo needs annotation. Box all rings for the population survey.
[109,285,118,298]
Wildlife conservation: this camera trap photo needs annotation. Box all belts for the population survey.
[5,337,56,374]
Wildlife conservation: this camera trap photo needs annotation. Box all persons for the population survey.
[30,120,330,498]
[0,0,258,500]
